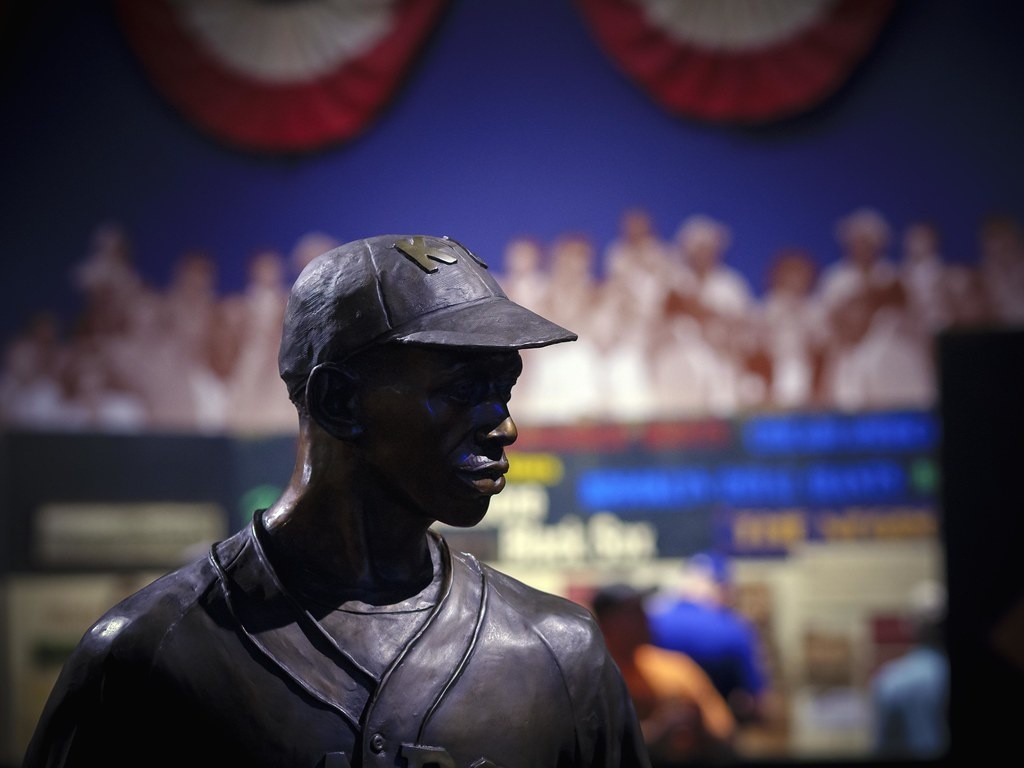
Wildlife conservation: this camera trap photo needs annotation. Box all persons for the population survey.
[0,196,1024,433]
[20,235,641,768]
[590,549,787,768]
[867,606,957,754]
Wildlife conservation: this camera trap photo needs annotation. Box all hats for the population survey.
[592,584,659,615]
